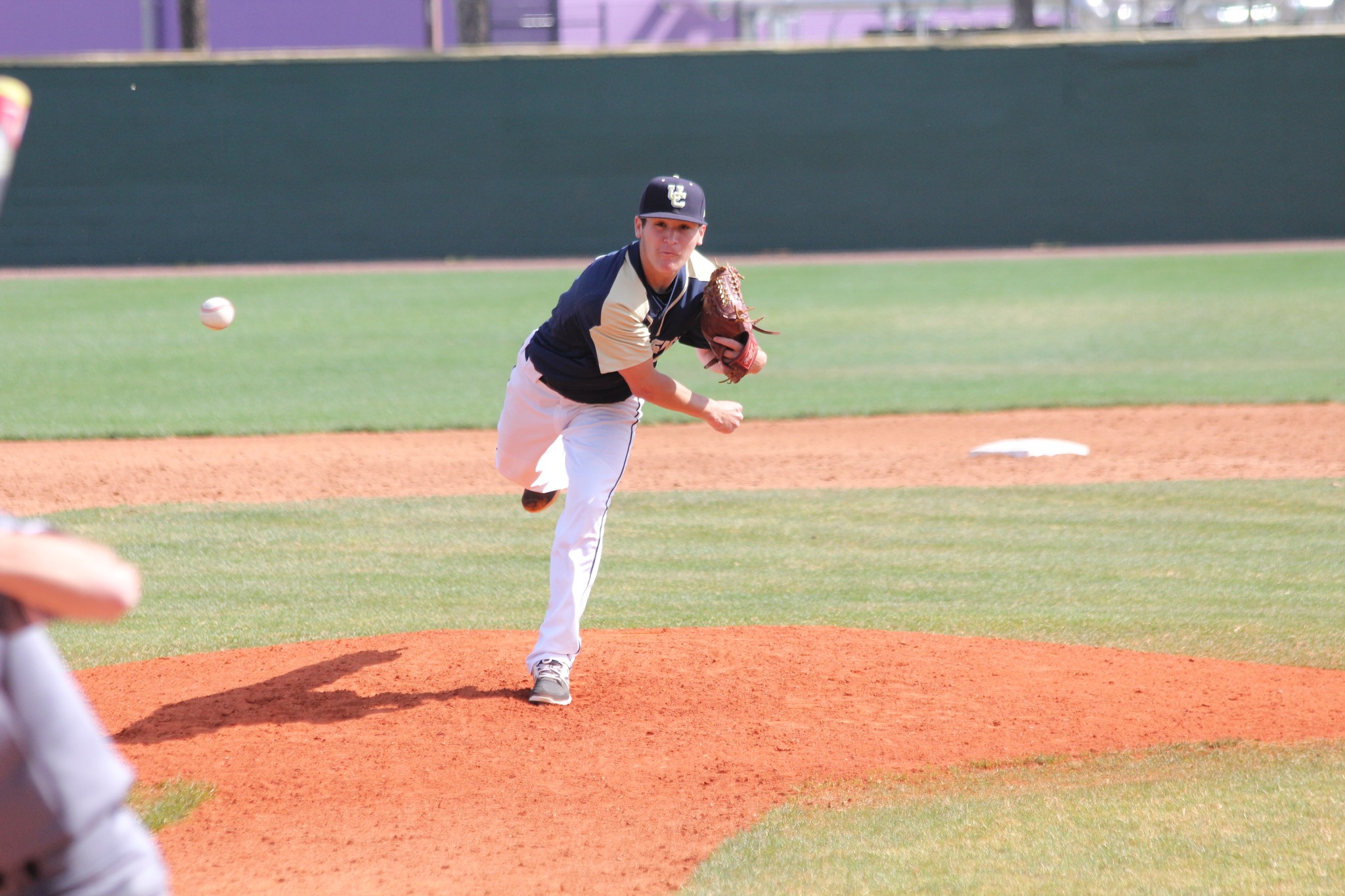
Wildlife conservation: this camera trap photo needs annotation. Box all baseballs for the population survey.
[198,297,235,331]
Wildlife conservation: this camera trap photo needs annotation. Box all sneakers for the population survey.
[530,657,572,705]
[522,485,562,512]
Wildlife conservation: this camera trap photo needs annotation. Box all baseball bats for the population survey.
[0,76,33,202]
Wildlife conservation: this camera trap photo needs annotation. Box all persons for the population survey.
[0,505,168,896]
[496,176,768,705]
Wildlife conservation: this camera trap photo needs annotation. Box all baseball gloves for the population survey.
[699,264,758,384]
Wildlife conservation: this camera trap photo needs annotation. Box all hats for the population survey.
[639,174,705,226]
